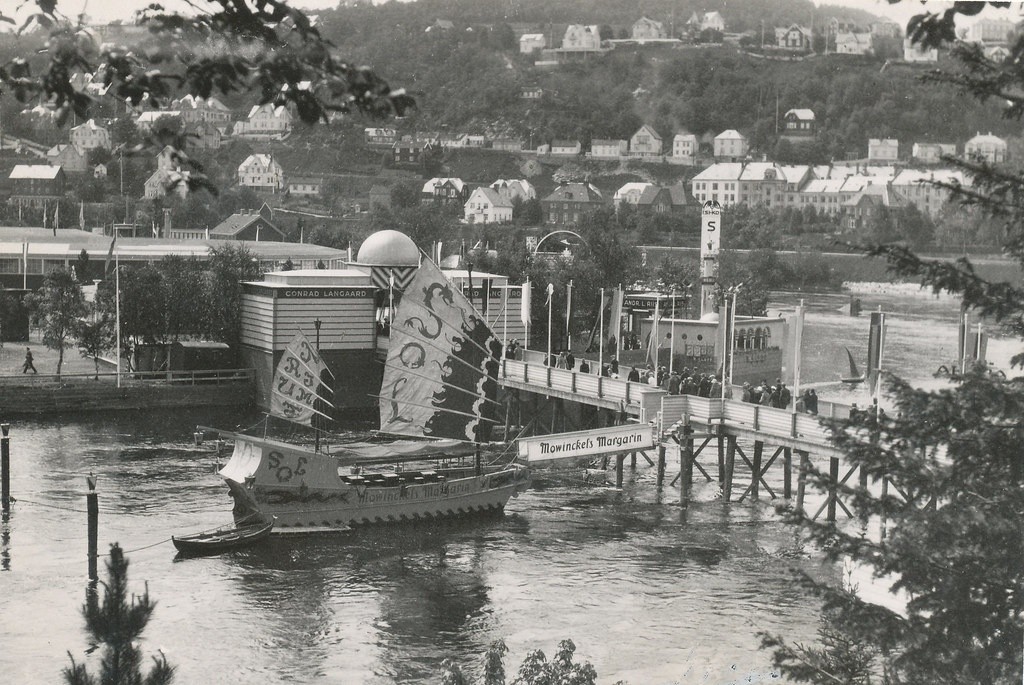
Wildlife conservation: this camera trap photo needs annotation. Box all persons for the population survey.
[505,338,523,361]
[796,388,818,416]
[740,378,791,410]
[580,358,589,374]
[22,347,37,375]
[597,355,618,378]
[627,364,732,399]
[543,350,575,370]
[849,398,903,435]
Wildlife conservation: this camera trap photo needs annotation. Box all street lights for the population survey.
[92,279,102,359]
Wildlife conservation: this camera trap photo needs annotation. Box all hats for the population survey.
[683,367,690,371]
[781,382,786,386]
[771,386,776,390]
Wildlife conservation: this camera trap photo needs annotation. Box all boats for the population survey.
[194,237,533,541]
[170,512,278,555]
